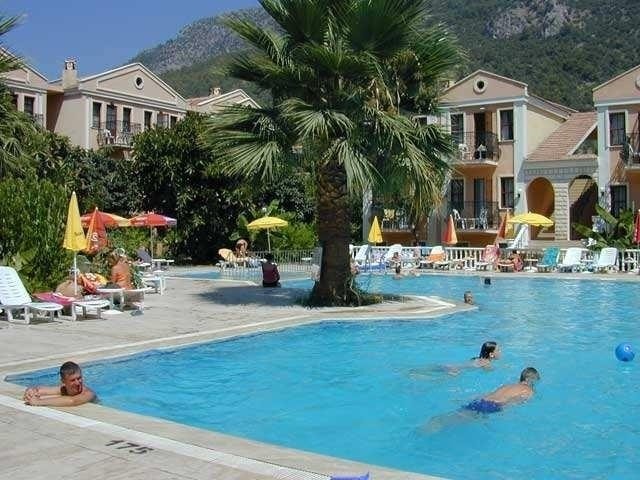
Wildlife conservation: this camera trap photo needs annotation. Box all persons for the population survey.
[393,253,421,281]
[417,368,540,436]
[22,361,100,407]
[235,237,248,258]
[463,291,472,304]
[406,342,501,383]
[484,277,491,287]
[349,255,359,276]
[262,254,282,287]
[499,248,518,264]
[100,245,151,290]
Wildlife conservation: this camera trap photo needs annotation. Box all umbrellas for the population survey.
[506,211,553,259]
[632,209,640,270]
[367,216,383,246]
[441,213,459,247]
[78,205,177,275]
[246,214,288,251]
[60,189,89,296]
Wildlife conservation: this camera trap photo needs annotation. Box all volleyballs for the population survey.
[616,344,634,362]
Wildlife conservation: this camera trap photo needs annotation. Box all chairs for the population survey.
[345,244,619,274]
[217,248,267,269]
[0,247,176,326]
